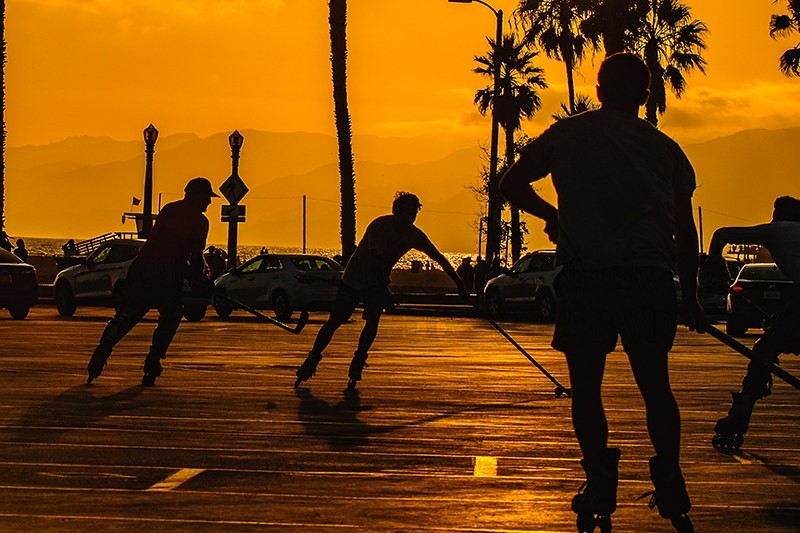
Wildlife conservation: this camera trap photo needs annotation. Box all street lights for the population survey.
[225,130,244,272]
[141,122,158,237]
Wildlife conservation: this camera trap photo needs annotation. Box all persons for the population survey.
[0,231,28,263]
[705,195,800,449]
[260,247,269,255]
[87,177,219,386]
[456,256,484,310]
[62,239,79,269]
[294,193,467,391]
[499,51,709,533]
[205,245,229,282]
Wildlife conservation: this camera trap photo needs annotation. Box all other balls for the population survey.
[555,387,563,395]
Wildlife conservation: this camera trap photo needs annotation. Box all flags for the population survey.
[132,197,141,205]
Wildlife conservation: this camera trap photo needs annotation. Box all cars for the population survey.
[673,260,745,324]
[212,251,358,319]
[725,262,791,337]
[0,246,39,319]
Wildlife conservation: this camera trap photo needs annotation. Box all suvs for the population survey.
[482,248,566,323]
[53,238,211,322]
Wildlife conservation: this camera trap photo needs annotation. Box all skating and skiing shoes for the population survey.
[349,352,368,390]
[712,392,755,453]
[635,456,695,533]
[295,351,322,388]
[142,347,163,386]
[572,449,621,532]
[87,344,112,384]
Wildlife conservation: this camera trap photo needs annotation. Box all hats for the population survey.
[185,178,220,197]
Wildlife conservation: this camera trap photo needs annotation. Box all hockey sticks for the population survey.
[730,287,774,322]
[467,295,572,396]
[705,323,800,389]
[211,288,310,334]
